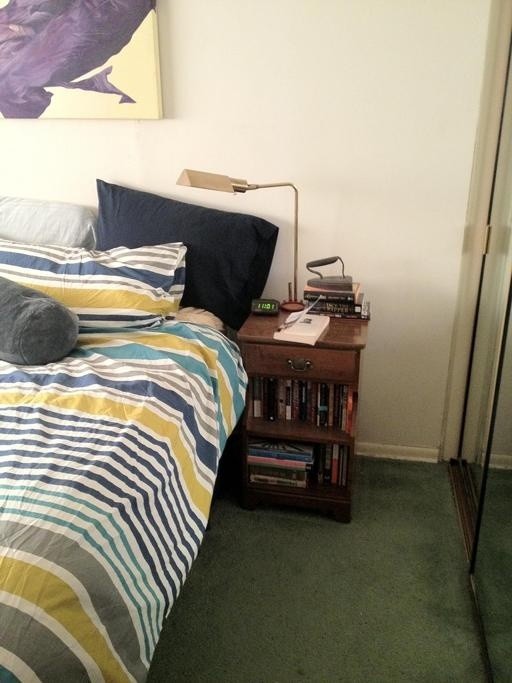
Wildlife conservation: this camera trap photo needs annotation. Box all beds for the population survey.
[2,197,250,682]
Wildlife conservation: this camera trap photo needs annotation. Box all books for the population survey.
[305,281,371,321]
[273,295,331,348]
[246,437,350,498]
[245,375,349,432]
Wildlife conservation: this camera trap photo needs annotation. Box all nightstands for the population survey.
[236,304,368,523]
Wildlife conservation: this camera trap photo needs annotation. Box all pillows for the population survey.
[1,276,80,365]
[95,178,278,330]
[1,237,186,330]
[3,197,99,251]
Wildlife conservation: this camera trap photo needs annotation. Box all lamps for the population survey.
[176,169,298,302]
